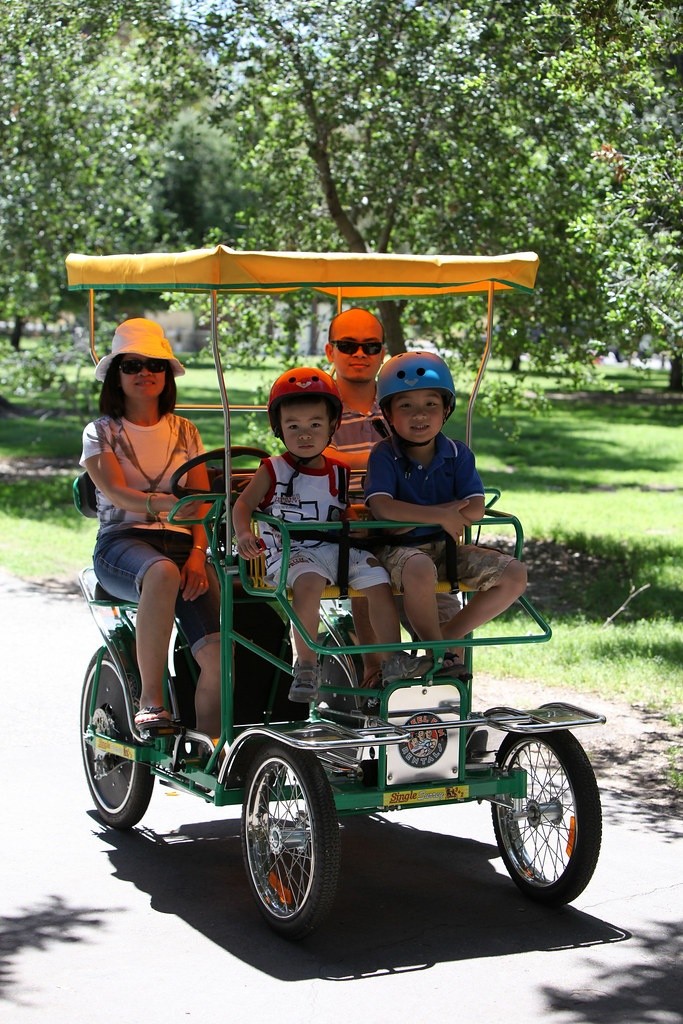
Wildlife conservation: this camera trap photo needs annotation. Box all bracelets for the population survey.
[191,545,207,555]
[146,493,161,515]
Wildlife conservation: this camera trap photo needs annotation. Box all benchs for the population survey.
[73,469,266,601]
[255,506,480,601]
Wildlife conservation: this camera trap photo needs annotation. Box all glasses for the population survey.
[330,340,383,356]
[119,358,166,374]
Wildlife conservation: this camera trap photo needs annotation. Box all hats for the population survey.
[93,318,185,383]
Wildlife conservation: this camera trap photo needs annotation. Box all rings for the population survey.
[199,582,204,585]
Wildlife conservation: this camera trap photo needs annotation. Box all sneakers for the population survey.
[381,650,433,687]
[288,659,322,703]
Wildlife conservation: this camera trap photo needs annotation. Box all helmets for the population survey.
[268,367,343,438]
[376,352,456,413]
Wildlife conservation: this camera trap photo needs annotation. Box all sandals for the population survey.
[434,653,473,682]
[134,706,170,729]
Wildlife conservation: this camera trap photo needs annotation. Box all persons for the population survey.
[232,367,386,708]
[78,317,235,740]
[325,308,395,689]
[364,351,528,674]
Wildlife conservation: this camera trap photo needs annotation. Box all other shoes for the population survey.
[360,666,384,716]
